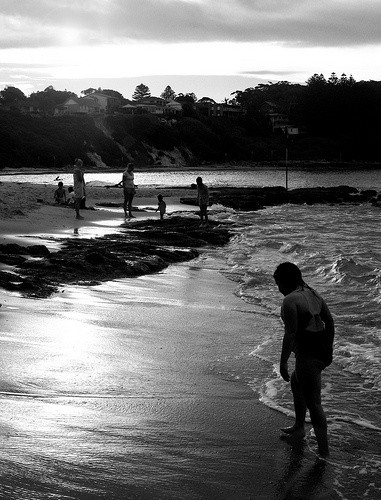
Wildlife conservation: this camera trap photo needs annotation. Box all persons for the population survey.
[66,187,95,210]
[156,194,166,219]
[73,161,85,220]
[273,262,335,458]
[122,163,137,218]
[196,177,209,228]
[54,182,66,205]
